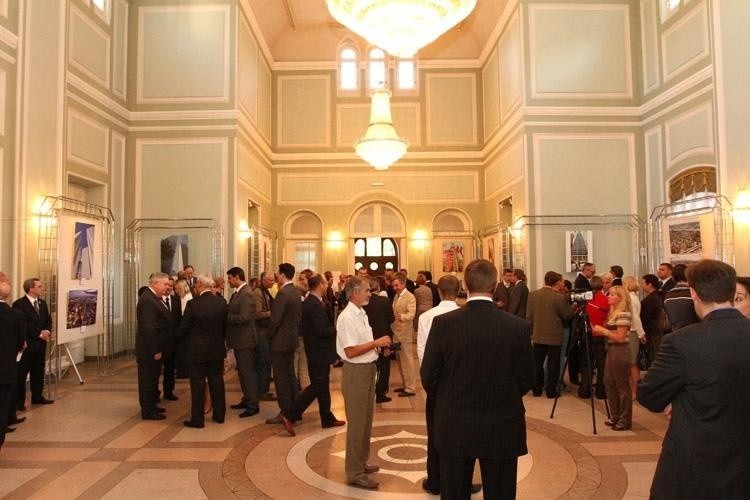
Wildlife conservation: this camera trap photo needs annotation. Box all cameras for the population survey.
[381,342,401,352]
[569,289,593,302]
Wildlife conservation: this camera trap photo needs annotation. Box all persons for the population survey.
[420,259,537,499]
[359,266,441,403]
[636,259,749,498]
[334,274,391,492]
[1,282,21,453]
[0,273,27,434]
[134,264,352,435]
[12,277,55,411]
[494,264,700,431]
[412,274,481,496]
[730,275,749,320]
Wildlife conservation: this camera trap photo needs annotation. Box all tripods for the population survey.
[550,303,610,434]
[44,341,84,385]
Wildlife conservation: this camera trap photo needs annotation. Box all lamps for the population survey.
[324,0,478,62]
[353,46,408,168]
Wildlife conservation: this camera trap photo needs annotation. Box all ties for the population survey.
[189,279,193,287]
[165,298,170,312]
[229,291,238,305]
[32,299,40,317]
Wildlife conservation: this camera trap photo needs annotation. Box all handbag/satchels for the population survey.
[635,338,653,372]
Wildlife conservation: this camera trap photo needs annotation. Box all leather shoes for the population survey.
[279,412,298,437]
[469,481,481,495]
[212,415,226,423]
[422,477,441,495]
[182,420,205,429]
[264,410,294,425]
[348,474,380,490]
[142,384,279,418]
[532,371,607,399]
[332,361,345,368]
[321,419,346,429]
[363,464,380,473]
[4,396,55,435]
[372,383,415,403]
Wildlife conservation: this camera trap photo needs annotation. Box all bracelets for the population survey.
[39,331,43,339]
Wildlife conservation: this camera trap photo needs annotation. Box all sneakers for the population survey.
[604,417,632,432]
